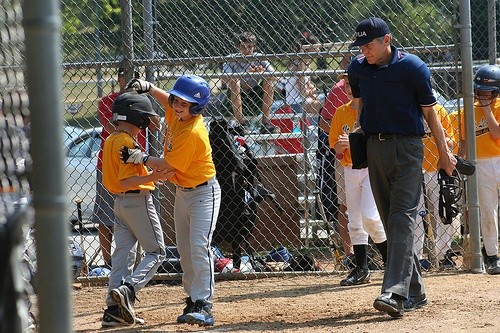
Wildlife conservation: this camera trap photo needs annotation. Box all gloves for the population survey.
[124,78,151,94]
[119,141,150,166]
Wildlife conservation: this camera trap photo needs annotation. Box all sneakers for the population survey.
[177,297,195,323]
[102,305,144,327]
[240,122,259,134]
[487,256,500,274]
[420,258,457,268]
[110,281,136,325]
[260,122,281,133]
[340,266,371,285]
[184,299,214,326]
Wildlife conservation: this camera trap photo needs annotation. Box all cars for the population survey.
[11,120,162,230]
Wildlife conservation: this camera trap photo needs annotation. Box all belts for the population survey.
[181,182,208,190]
[121,190,151,194]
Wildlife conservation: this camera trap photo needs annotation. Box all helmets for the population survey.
[112,91,159,129]
[168,74,211,116]
[474,65,500,100]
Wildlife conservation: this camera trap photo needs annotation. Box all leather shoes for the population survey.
[373,293,405,318]
[404,293,427,311]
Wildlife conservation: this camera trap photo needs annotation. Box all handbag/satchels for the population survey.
[349,132,368,169]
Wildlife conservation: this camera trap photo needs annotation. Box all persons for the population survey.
[453,65,500,277]
[322,16,458,317]
[285,33,328,113]
[222,34,283,137]
[0,88,40,307]
[125,72,223,327]
[92,62,162,275]
[204,115,264,274]
[102,89,175,330]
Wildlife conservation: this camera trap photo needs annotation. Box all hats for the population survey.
[351,17,390,47]
[118,65,140,77]
[338,72,349,78]
